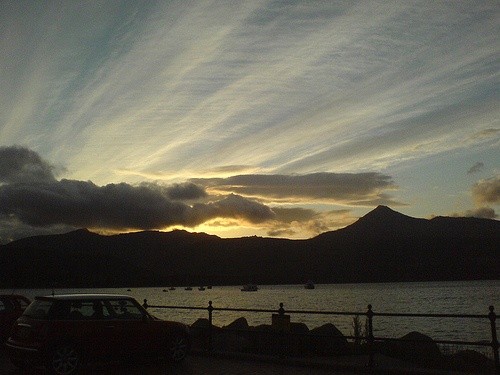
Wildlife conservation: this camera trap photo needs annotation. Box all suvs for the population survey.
[0,295,33,343]
[6,293,192,373]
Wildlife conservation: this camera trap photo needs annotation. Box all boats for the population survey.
[240,284,257,292]
[304,280,315,289]
[169,283,213,291]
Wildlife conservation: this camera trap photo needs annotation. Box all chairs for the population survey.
[31,309,47,319]
[70,311,84,320]
